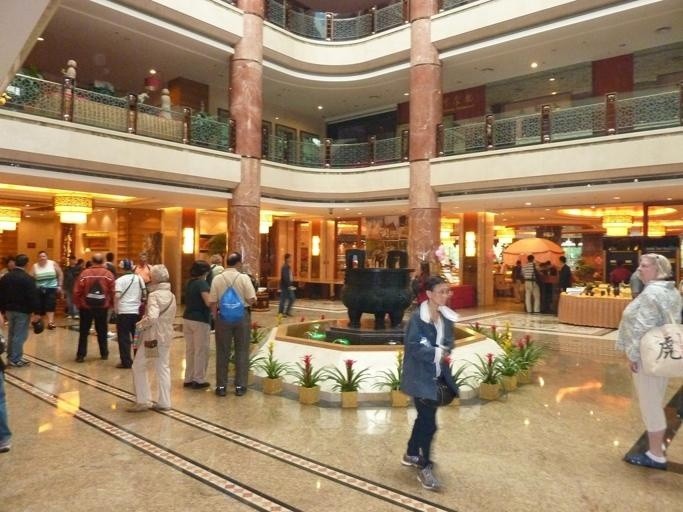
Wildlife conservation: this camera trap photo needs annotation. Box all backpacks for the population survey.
[86,280,107,307]
[219,287,244,325]
[639,323,683,379]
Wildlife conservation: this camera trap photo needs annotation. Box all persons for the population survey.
[521,255,541,313]
[3,256,15,275]
[629,270,643,299]
[511,260,524,304]
[182,260,211,391]
[615,253,683,470]
[210,254,225,279]
[65,256,80,320]
[209,251,257,396]
[132,254,153,286]
[0,312,13,455]
[558,256,571,292]
[104,253,116,274]
[77,259,84,267]
[114,259,147,369]
[30,251,64,329]
[608,257,632,284]
[71,253,115,363]
[277,253,296,318]
[0,253,44,367]
[545,260,556,276]
[398,277,461,491]
[122,264,176,412]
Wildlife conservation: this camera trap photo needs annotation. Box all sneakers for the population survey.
[48,320,56,328]
[75,356,83,361]
[281,312,287,317]
[192,381,210,390]
[74,315,79,319]
[215,387,225,394]
[101,354,107,359]
[401,453,434,470]
[126,405,147,412]
[8,360,30,368]
[287,313,294,317]
[116,364,128,368]
[68,315,72,318]
[0,438,10,451]
[184,383,191,386]
[152,403,170,411]
[235,387,247,395]
[416,465,440,491]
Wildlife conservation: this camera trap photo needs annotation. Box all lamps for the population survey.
[643,224,667,237]
[311,235,321,255]
[52,195,93,225]
[259,214,274,234]
[1,204,21,231]
[465,232,477,257]
[496,229,515,243]
[603,215,633,236]
[145,69,161,91]
[183,228,195,255]
[440,218,461,240]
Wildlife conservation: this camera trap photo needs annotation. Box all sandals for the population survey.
[624,451,667,470]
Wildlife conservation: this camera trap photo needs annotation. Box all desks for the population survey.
[269,274,342,299]
[556,291,633,330]
[449,281,474,309]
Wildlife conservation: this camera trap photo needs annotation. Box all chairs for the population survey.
[494,273,515,298]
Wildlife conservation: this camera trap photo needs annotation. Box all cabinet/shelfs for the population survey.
[641,236,680,287]
[603,236,640,286]
[339,235,366,282]
[81,231,111,253]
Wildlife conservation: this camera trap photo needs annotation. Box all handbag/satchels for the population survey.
[531,263,544,288]
[109,274,135,323]
[640,284,683,378]
[419,376,455,408]
[106,263,115,274]
[144,293,174,357]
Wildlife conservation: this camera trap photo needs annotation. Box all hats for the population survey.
[117,259,135,268]
[31,319,44,334]
